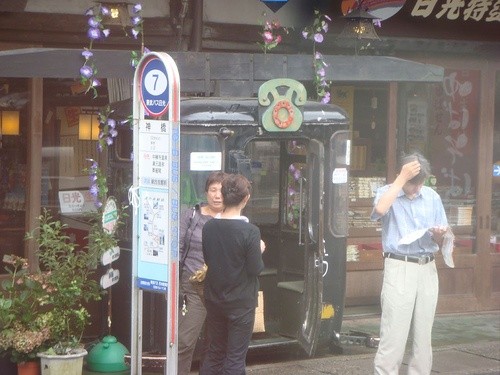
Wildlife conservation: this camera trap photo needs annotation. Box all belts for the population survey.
[383,251,436,264]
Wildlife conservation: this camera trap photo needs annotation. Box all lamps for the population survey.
[335,5,381,56]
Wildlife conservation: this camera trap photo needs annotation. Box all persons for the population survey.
[369,153,450,375]
[179,173,266,375]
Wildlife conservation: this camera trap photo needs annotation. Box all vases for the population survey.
[16,349,88,375]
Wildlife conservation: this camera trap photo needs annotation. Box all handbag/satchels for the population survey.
[190,263,209,302]
[160,293,187,316]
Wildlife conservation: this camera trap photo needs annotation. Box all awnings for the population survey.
[1,42,444,83]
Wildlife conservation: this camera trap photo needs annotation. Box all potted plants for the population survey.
[19,204,129,356]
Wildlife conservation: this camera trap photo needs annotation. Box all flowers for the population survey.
[0,255,57,351]
